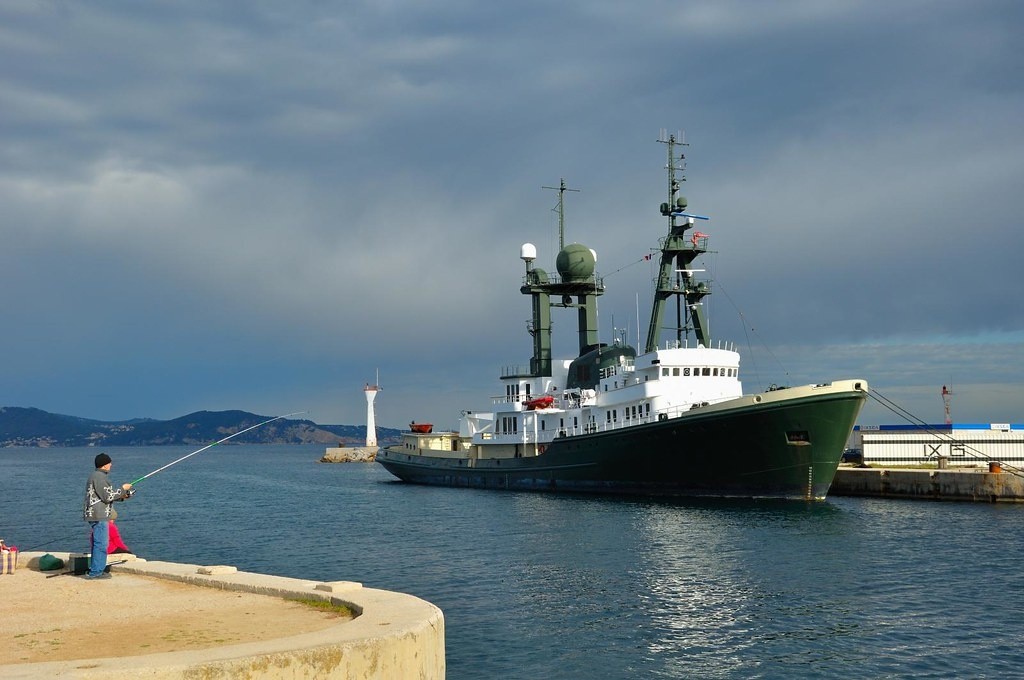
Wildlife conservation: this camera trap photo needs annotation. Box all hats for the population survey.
[95,454,111,468]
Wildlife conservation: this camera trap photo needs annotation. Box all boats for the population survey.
[376,128,870,499]
[409,423,433,432]
[522,396,554,410]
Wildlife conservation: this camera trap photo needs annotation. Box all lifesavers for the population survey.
[658,413,668,422]
[539,445,545,453]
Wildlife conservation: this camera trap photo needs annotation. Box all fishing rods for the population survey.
[130,411,307,485]
[46,559,129,578]
[18,532,84,553]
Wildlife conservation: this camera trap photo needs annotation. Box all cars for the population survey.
[840,448,863,462]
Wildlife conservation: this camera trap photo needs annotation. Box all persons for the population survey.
[84,453,136,579]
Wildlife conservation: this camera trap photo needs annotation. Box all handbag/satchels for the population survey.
[0,544,17,574]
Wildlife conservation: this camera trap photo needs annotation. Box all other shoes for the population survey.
[89,572,112,580]
